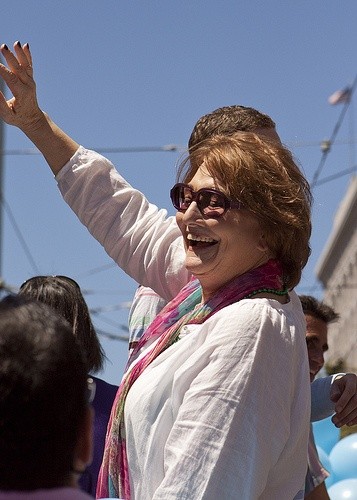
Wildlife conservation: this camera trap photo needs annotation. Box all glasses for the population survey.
[170,182,238,218]
[20,275,80,290]
